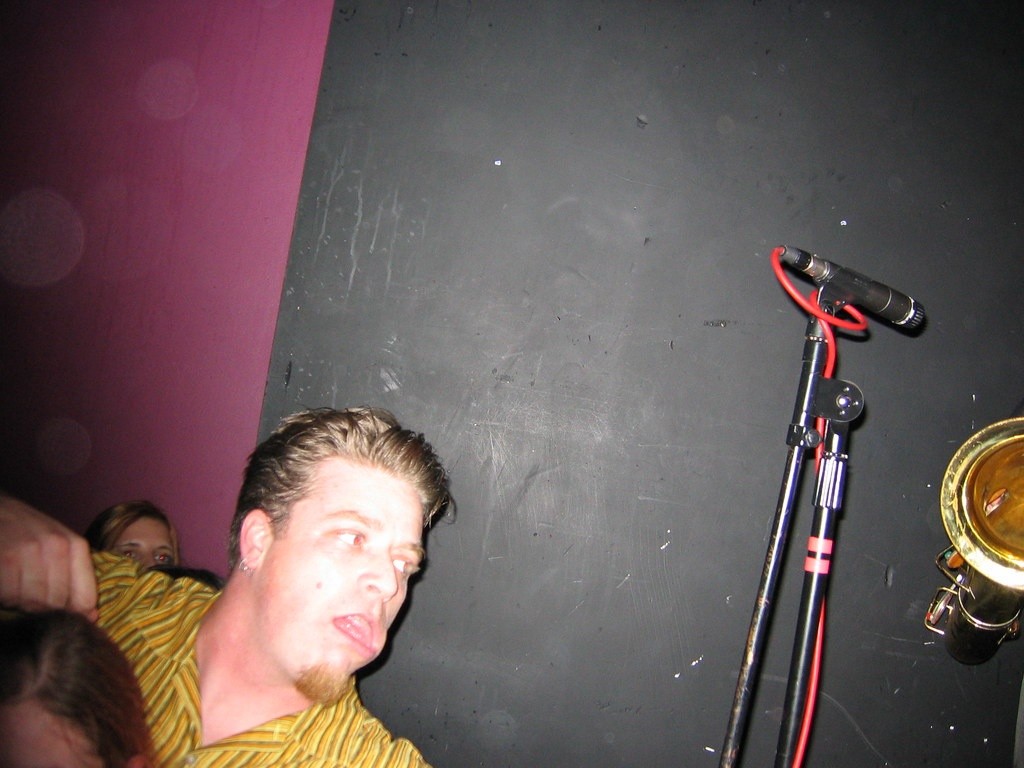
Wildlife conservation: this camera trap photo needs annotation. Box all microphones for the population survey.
[778,244,925,329]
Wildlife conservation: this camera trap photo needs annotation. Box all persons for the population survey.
[0,407,458,768]
[1,607,154,768]
[87,499,179,569]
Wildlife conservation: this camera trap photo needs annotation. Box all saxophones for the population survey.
[925,397,1024,667]
